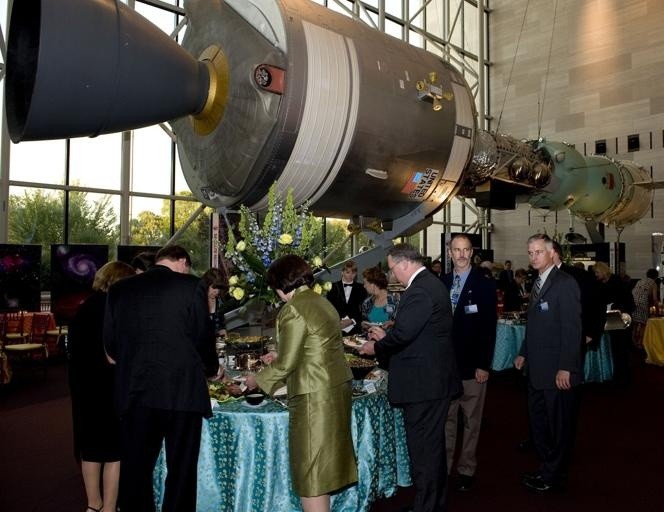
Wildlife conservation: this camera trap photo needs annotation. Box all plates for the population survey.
[241,400,269,409]
[344,340,367,348]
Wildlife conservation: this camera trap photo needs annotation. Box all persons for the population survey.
[132,253,155,274]
[592,261,635,347]
[550,240,594,383]
[361,266,402,341]
[65,261,136,512]
[358,242,464,512]
[514,234,582,494]
[633,268,660,352]
[244,255,359,511]
[201,269,230,339]
[326,259,370,336]
[500,260,515,311]
[503,269,530,313]
[102,245,219,512]
[430,260,442,275]
[438,234,498,492]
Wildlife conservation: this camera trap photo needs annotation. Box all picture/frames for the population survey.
[0,244,42,315]
[117,245,165,271]
[50,244,109,326]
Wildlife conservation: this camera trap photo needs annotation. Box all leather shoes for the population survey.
[464,474,481,497]
[451,476,470,496]
[527,481,559,498]
[524,476,549,490]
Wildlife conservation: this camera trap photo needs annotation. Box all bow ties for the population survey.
[342,281,352,288]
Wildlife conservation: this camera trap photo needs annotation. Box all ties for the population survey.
[529,275,541,308]
[449,273,461,316]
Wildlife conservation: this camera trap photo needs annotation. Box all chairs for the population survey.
[4,308,29,358]
[4,313,51,373]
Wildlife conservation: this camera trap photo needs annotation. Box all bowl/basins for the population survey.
[348,359,380,380]
[244,393,264,406]
